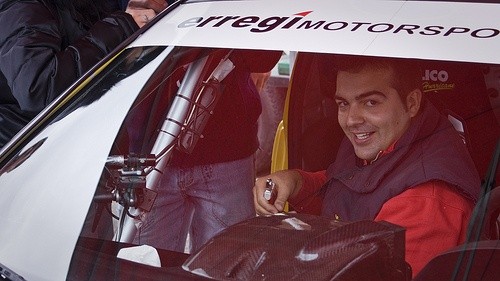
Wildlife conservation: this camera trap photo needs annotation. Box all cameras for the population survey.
[106,152,156,207]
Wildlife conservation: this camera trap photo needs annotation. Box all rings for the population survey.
[144,14,149,24]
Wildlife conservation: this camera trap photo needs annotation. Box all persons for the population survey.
[118,49,285,253]
[252,53,483,281]
[0,0,157,147]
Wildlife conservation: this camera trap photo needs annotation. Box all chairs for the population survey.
[410,59,500,192]
